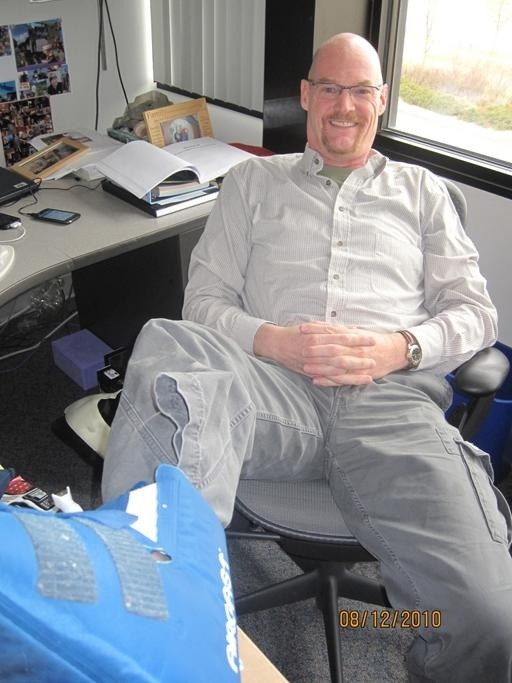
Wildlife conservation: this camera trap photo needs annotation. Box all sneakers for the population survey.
[0,464,244,683]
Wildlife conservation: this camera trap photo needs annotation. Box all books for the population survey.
[26,114,258,217]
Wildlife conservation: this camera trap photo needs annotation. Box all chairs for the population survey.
[231,163,512,681]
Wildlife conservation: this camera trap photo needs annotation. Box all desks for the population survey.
[1,128,229,309]
[235,623,297,683]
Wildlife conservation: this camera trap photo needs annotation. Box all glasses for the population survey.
[308,80,383,101]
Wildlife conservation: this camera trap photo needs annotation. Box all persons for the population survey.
[47,72,65,95]
[101,32,512,682]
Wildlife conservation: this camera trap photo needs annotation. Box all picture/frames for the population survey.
[11,136,90,183]
[141,96,215,148]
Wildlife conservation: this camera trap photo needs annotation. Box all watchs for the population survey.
[397,329,421,373]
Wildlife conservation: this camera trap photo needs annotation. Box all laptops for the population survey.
[0,166,37,204]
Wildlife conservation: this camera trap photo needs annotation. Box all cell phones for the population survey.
[35,208,81,224]
[0,212,21,230]
